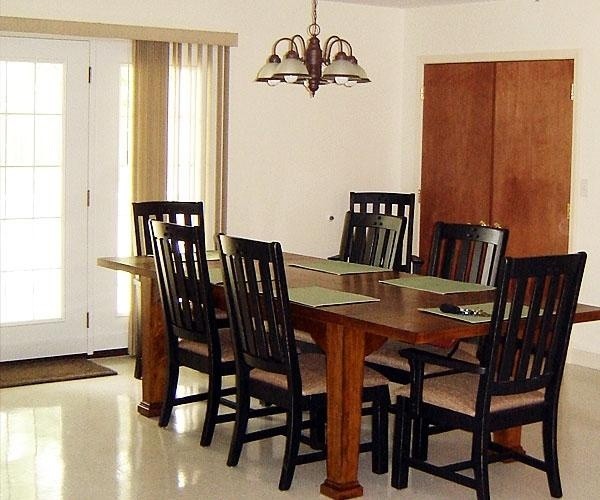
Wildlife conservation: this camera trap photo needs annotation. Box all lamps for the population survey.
[255,0,372,98]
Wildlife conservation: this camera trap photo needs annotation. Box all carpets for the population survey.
[0,355,118,388]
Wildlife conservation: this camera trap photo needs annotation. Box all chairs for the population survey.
[390,252,588,500]
[255,212,407,406]
[131,201,207,381]
[328,191,424,275]
[364,221,510,385]
[213,231,391,490]
[147,218,325,451]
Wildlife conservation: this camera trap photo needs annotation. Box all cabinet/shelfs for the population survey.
[418,58,575,358]
